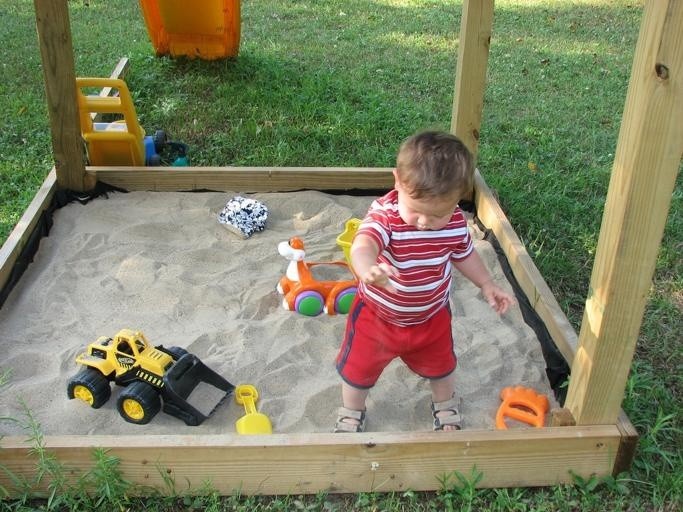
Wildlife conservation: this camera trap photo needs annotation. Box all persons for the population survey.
[327,128,518,439]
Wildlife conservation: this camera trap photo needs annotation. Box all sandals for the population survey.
[333,406,367,433]
[430,391,462,431]
